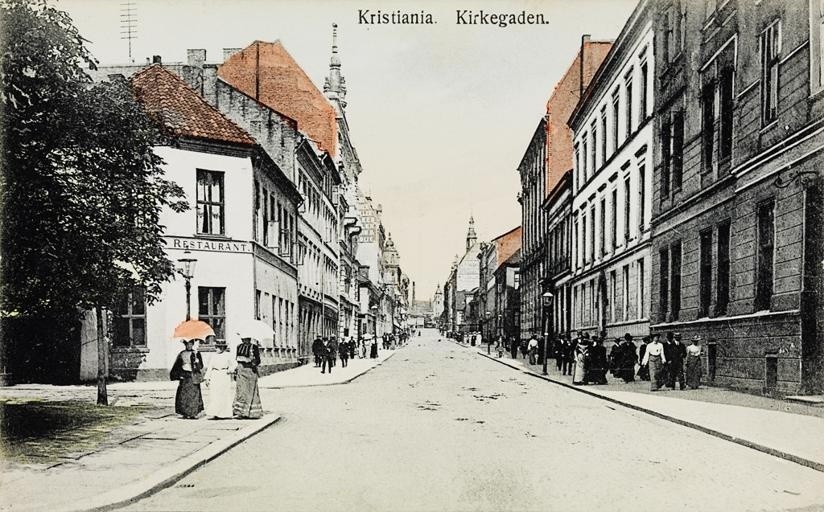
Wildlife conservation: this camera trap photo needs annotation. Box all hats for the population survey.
[642,331,701,341]
[215,338,227,347]
[317,333,336,339]
[350,336,354,339]
[577,330,634,345]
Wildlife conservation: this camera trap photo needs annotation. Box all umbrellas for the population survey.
[173,319,217,341]
[233,318,275,340]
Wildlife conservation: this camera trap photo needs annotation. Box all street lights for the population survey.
[372,305,379,345]
[485,311,490,354]
[177,245,198,321]
[542,286,554,376]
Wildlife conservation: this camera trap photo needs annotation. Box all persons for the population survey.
[205,338,238,419]
[170,338,206,420]
[234,338,265,420]
[307,326,410,375]
[446,329,709,395]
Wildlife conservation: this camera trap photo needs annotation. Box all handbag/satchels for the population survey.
[192,371,205,383]
